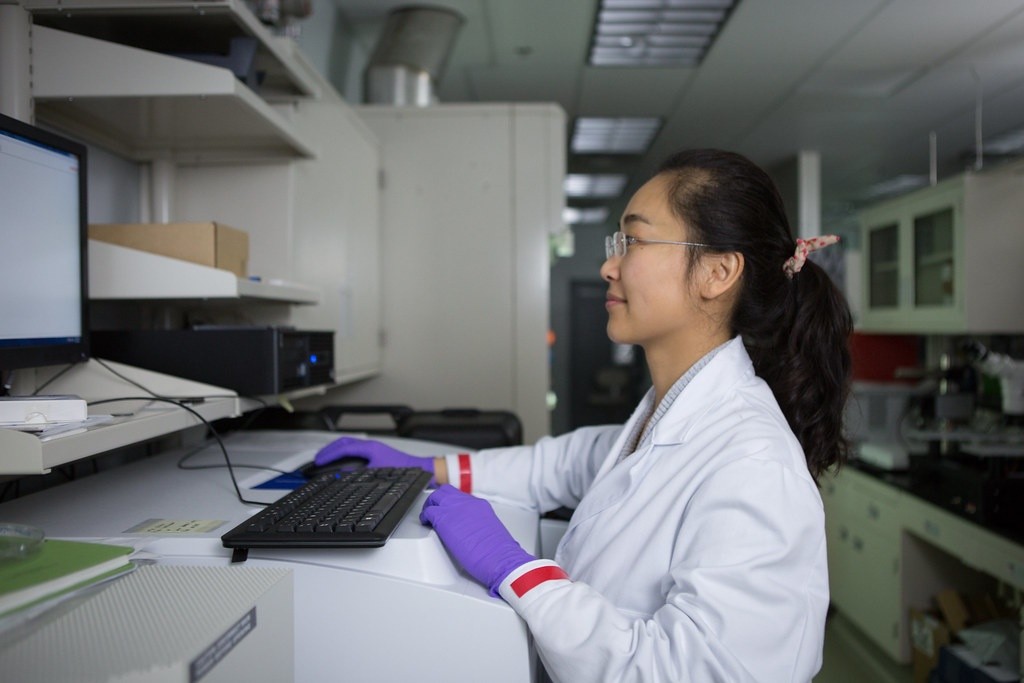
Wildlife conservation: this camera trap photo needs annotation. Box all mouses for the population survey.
[305,456,368,477]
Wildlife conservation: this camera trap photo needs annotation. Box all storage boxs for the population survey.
[85,220,250,278]
[912,587,1000,683]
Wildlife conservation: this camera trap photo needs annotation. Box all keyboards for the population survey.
[221,466,433,564]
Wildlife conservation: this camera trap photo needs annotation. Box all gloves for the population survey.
[315,437,440,489]
[419,484,538,599]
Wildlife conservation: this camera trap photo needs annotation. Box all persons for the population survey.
[315,149,853,683]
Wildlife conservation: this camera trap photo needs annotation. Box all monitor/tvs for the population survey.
[0,113,90,368]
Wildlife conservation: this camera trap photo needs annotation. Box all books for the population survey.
[0,537,137,620]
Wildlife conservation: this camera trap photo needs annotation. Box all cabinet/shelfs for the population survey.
[0,0,382,475]
[852,164,1023,334]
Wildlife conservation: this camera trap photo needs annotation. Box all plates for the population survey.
[0,524,46,560]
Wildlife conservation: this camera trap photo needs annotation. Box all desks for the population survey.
[814,460,1024,666]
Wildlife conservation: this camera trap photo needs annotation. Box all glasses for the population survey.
[605,232,734,259]
